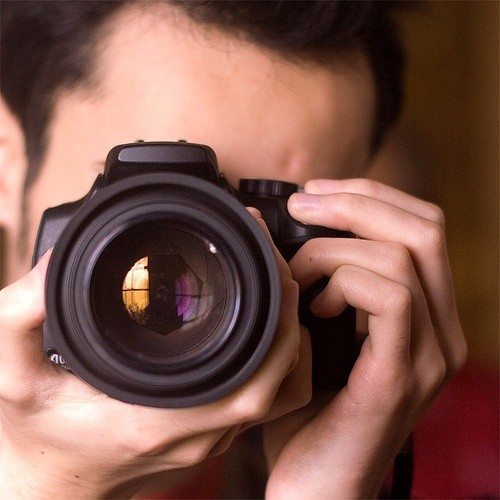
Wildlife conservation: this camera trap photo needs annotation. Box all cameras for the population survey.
[32,136,354,410]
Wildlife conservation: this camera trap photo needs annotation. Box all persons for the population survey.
[0,1,467,500]
